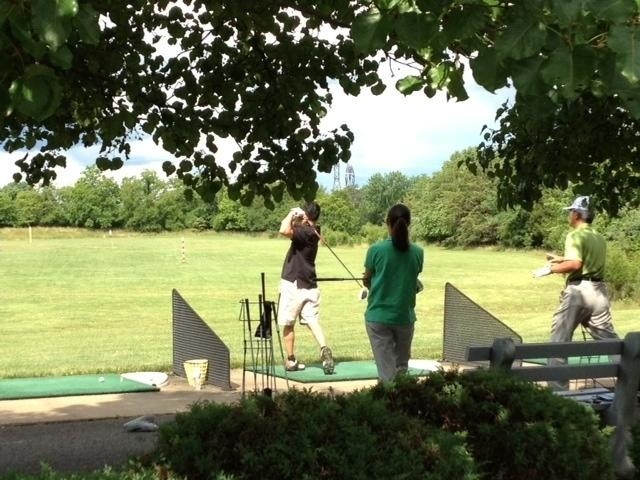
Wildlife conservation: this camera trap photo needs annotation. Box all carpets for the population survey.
[0,370,161,401]
[523,354,612,368]
[244,351,435,385]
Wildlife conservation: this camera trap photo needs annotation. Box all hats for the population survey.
[563,196,590,211]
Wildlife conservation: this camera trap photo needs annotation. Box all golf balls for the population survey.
[98,377,105,383]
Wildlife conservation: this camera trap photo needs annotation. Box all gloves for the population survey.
[545,253,565,263]
[533,268,551,278]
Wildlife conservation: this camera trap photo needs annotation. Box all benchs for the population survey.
[463,332,640,442]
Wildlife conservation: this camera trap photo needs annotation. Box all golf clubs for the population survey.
[245,272,289,404]
[305,220,368,300]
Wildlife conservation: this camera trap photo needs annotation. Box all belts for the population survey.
[569,277,602,282]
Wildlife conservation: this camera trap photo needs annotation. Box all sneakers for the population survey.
[320,346,334,375]
[286,360,306,371]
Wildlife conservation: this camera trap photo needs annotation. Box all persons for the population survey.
[538,192,624,390]
[357,203,425,382]
[275,197,336,376]
